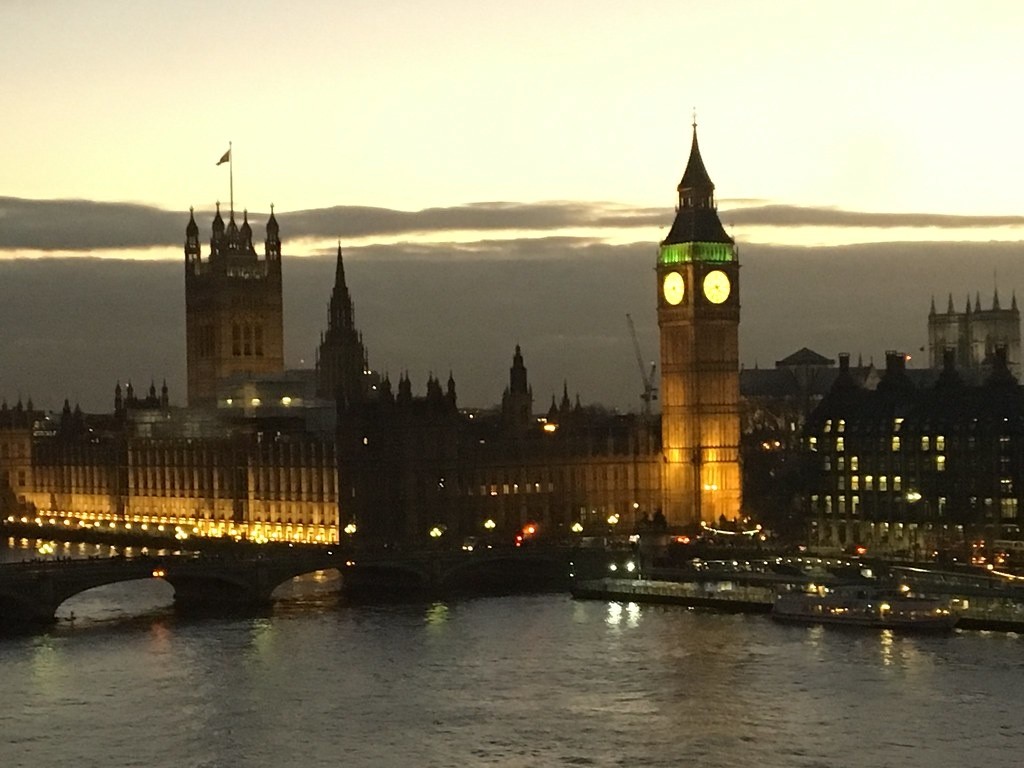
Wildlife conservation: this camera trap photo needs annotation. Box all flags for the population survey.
[216,149,231,166]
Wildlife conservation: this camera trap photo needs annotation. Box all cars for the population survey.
[645,530,681,546]
[357,543,401,559]
[560,529,607,548]
[191,541,243,562]
[695,529,732,544]
[568,521,616,537]
[462,535,496,552]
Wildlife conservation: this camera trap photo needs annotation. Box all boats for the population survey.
[771,573,961,633]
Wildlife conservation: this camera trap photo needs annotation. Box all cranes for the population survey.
[625,313,659,413]
